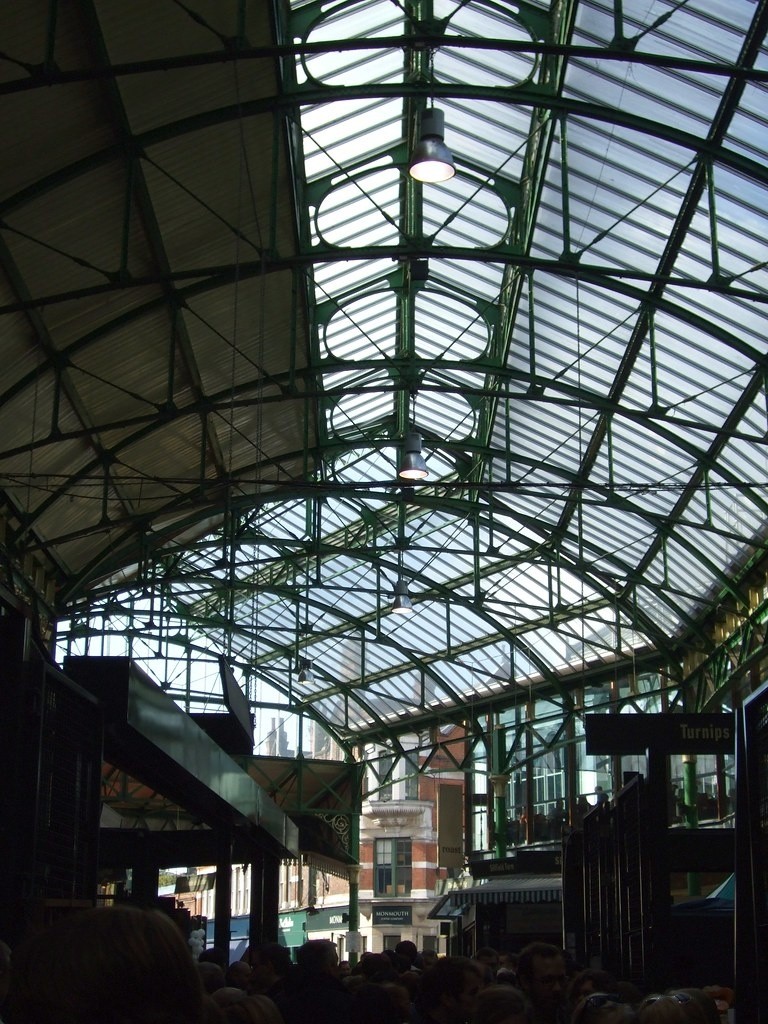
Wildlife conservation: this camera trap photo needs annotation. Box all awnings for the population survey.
[427,895,469,920]
[451,874,578,907]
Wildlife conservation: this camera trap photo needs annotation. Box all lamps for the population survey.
[407,19,457,183]
[297,627,315,684]
[398,380,430,480]
[391,542,413,614]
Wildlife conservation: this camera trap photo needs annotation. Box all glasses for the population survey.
[641,990,695,1007]
[524,974,565,988]
[580,993,628,1015]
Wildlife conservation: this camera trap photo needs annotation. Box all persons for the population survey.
[505,786,609,844]
[672,784,682,824]
[0,906,731,1023]
